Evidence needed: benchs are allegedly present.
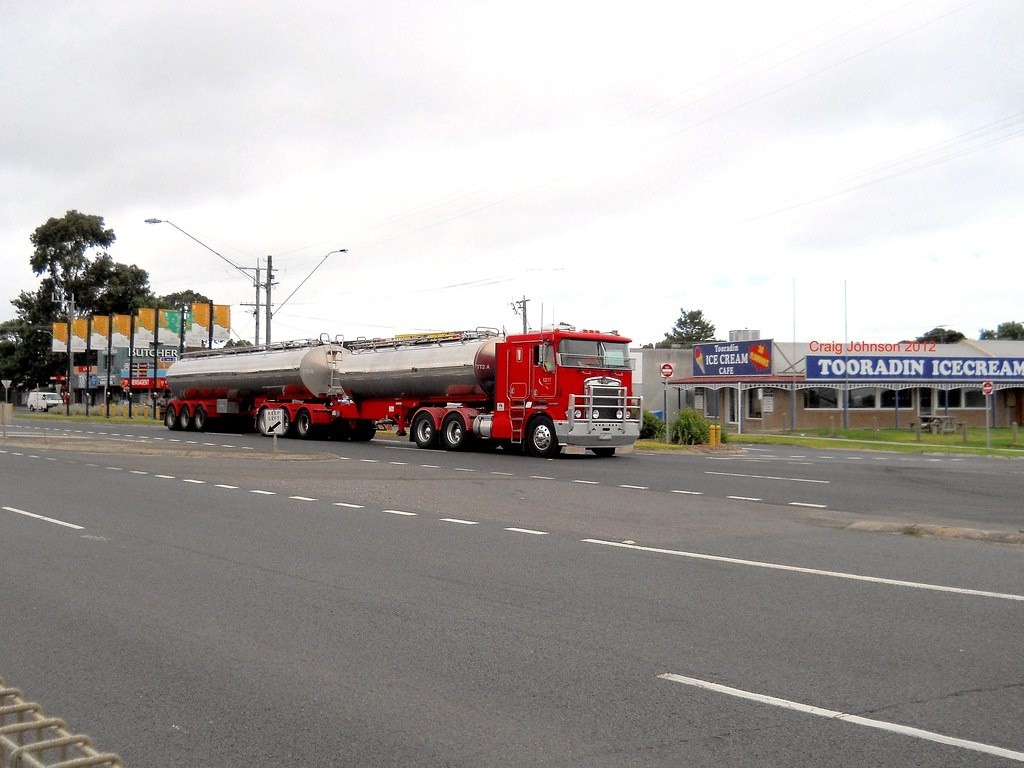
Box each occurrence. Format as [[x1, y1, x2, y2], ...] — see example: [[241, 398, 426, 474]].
[[918, 420, 965, 435]]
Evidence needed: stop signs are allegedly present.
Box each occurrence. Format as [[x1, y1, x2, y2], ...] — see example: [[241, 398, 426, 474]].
[[982, 382, 993, 395], [661, 363, 674, 377]]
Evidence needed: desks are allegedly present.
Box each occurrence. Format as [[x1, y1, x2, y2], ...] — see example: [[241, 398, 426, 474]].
[[919, 415, 956, 435]]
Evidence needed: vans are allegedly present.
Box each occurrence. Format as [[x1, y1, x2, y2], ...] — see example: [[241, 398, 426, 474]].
[[26, 391, 64, 413]]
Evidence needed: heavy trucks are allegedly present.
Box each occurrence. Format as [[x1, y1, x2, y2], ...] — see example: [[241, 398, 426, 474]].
[[160, 323, 644, 459]]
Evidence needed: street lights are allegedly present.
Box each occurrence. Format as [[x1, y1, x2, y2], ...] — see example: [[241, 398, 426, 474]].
[[145, 218, 260, 348], [264, 248, 347, 344]]
